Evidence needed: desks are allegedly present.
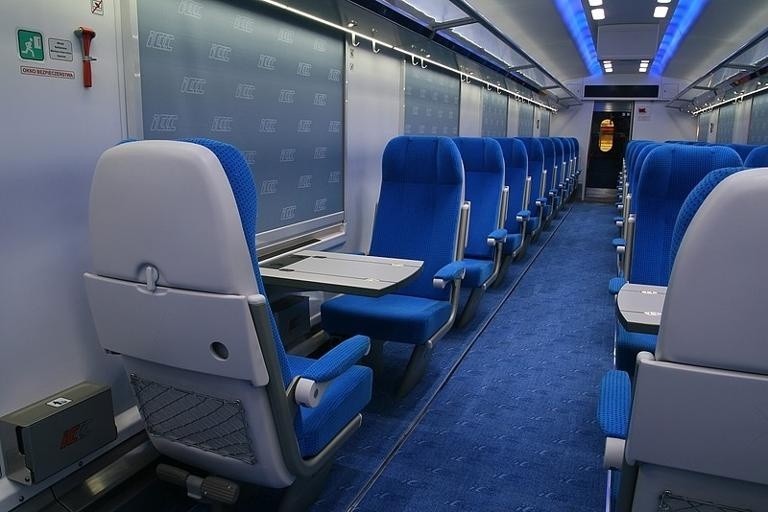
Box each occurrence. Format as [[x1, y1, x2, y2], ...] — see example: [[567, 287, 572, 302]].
[[258, 249, 425, 299]]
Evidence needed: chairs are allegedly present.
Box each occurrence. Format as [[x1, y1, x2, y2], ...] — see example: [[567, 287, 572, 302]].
[[457, 136, 580, 328], [319, 134, 472, 404], [594, 139, 767, 511], [81, 137, 374, 510]]
